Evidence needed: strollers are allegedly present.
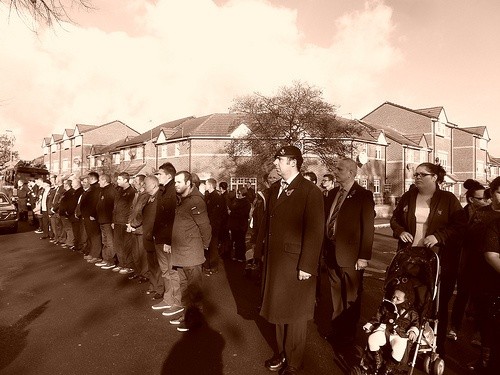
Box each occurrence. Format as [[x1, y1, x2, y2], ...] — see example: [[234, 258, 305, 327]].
[[350, 246, 445, 375]]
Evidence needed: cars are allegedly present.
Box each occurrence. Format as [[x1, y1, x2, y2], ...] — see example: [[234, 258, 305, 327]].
[[0, 192, 18, 233]]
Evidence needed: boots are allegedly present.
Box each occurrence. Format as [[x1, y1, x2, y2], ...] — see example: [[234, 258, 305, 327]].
[[370, 350, 386, 375], [385, 358, 401, 375]]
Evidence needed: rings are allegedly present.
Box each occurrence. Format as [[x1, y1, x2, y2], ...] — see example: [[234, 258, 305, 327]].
[[428, 242, 430, 244]]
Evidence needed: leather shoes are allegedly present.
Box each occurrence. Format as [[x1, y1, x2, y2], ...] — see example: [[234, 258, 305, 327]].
[[264, 352, 287, 372], [282, 366, 298, 375]]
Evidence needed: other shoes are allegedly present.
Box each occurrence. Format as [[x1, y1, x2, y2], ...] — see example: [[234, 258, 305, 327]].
[[144, 289, 156, 294], [176, 322, 189, 331], [138, 278, 149, 283], [204, 268, 219, 277], [34, 230, 134, 274], [169, 315, 185, 324], [152, 301, 171, 310], [161, 307, 184, 316], [153, 294, 163, 301], [127, 276, 140, 279], [246, 263, 259, 270]]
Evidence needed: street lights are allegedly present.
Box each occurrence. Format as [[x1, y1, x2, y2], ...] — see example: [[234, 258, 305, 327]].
[[6, 130, 13, 182]]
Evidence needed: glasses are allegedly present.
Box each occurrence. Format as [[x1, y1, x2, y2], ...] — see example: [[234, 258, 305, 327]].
[[473, 196, 484, 200], [414, 173, 434, 178], [322, 180, 329, 182]]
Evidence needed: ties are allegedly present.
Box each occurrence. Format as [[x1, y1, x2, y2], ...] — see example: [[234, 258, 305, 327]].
[[328, 188, 346, 240], [277, 181, 288, 198]]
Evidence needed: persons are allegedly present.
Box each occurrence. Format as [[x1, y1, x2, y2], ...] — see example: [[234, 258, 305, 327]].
[[363, 287, 419, 375], [303, 172, 317, 185], [126, 174, 149, 284], [259, 146, 325, 375], [321, 173, 334, 195], [191, 173, 269, 269], [390, 164, 467, 358], [30, 172, 102, 263], [94, 174, 117, 269], [112, 172, 134, 273], [153, 163, 185, 316], [320, 158, 376, 345], [451, 175, 500, 370], [16, 179, 31, 222], [170, 171, 211, 332], [141, 176, 165, 300], [268, 168, 281, 186]]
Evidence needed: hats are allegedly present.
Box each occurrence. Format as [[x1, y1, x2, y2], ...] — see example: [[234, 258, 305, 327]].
[[274, 146, 301, 158]]
